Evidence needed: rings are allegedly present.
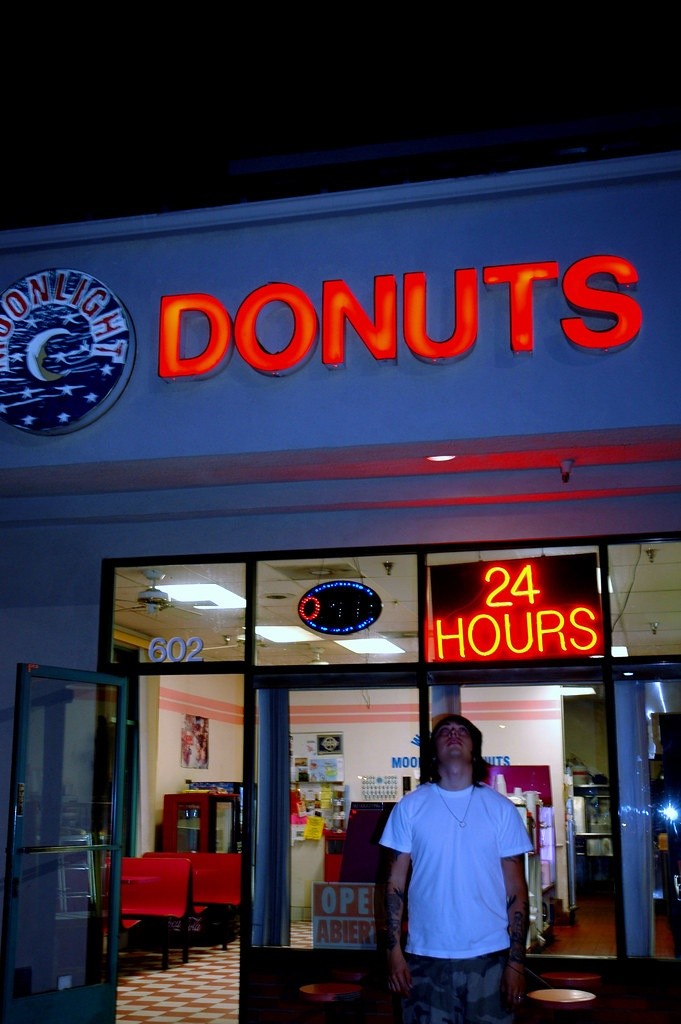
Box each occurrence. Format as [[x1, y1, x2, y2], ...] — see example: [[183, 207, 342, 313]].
[[515, 997, 522, 1000]]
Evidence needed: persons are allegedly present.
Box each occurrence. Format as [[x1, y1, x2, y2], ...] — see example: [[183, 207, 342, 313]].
[[379, 714, 534, 1024]]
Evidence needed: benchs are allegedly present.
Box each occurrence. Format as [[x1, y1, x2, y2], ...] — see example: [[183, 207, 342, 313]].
[[98, 852, 242, 969]]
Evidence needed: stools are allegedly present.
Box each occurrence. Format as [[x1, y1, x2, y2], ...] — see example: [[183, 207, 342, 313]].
[[300, 967, 373, 1024], [525, 971, 602, 1024]]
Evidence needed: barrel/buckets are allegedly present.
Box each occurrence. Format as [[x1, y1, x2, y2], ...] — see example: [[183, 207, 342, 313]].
[[571, 765, 587, 785]]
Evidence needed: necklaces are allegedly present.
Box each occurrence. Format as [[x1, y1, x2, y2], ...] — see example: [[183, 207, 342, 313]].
[[436, 783, 475, 828]]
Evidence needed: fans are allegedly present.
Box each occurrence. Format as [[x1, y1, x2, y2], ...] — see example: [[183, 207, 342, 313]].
[[114, 568, 219, 616]]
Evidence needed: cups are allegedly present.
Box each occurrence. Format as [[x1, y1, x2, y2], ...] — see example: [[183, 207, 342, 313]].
[[494, 774, 508, 797]]
